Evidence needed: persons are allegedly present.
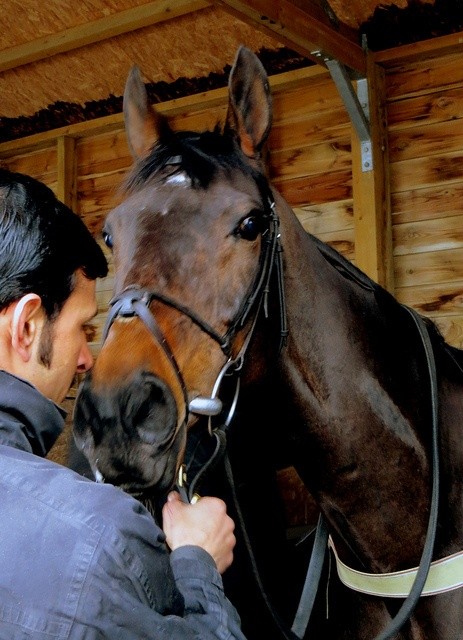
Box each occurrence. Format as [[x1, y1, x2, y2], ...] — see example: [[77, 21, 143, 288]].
[[0, 167, 247, 638]]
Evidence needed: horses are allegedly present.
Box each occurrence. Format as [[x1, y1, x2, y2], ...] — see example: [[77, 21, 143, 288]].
[[68, 41, 463, 640]]
